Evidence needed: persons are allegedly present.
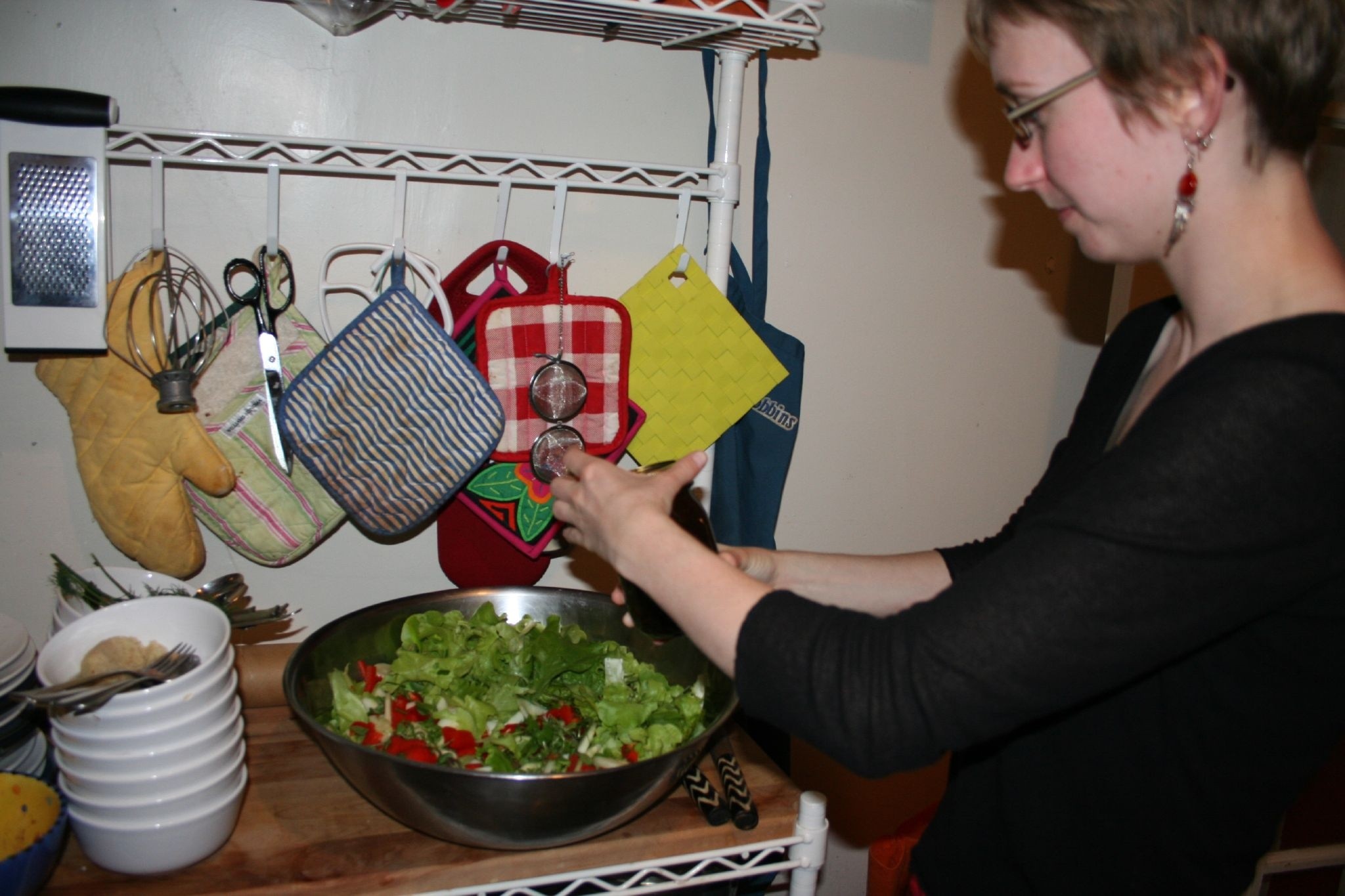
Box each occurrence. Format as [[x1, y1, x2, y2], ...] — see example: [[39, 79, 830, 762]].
[[551, 1, 1345, 896]]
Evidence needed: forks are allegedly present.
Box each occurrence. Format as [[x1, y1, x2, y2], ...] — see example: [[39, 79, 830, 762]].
[[11, 641, 195, 701]]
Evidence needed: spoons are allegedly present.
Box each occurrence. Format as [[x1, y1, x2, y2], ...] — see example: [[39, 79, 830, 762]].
[[216, 584, 249, 605], [192, 573, 244, 600], [72, 653, 201, 715]]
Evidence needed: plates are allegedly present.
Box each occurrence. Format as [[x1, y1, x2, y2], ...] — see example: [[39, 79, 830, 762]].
[[0, 731, 49, 782], [0, 612, 39, 726]]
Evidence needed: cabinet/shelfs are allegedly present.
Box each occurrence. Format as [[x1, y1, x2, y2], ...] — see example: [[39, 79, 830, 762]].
[[41, 0, 833, 896]]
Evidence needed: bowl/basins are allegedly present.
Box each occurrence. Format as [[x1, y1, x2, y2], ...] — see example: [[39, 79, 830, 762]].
[[284, 583, 740, 846], [0, 772, 66, 896], [37, 570, 251, 876]]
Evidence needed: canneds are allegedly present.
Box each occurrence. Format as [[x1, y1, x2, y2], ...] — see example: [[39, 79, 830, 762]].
[[618, 458, 722, 643]]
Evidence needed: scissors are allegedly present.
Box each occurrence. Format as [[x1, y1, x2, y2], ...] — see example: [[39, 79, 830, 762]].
[[226, 245, 294, 476]]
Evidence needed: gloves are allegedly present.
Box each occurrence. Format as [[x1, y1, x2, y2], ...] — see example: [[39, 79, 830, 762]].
[[33, 257, 235, 581]]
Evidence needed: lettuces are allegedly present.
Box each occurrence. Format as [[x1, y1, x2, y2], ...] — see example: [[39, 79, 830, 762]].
[[324, 601, 706, 772]]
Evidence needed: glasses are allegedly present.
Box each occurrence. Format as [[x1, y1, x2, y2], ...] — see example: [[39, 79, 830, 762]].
[[999, 60, 1114, 150]]
